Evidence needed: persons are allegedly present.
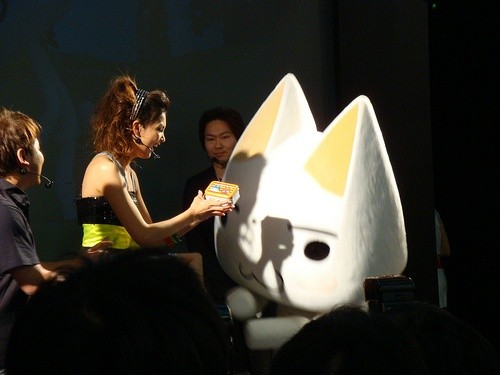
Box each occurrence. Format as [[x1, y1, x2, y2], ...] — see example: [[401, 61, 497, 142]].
[[0, 110, 65, 369], [183, 106, 245, 295], [6, 248, 236, 375], [75, 77, 233, 283], [269, 303, 500, 375]]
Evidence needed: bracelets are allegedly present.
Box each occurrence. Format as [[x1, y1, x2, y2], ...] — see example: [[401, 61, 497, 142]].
[[170, 233, 182, 243], [165, 237, 173, 246]]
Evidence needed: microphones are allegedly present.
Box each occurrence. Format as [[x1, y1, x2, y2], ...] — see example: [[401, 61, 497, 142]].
[[134, 138, 161, 161], [18, 168, 53, 189]]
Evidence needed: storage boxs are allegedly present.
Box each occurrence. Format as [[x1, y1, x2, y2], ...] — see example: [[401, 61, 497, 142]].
[[205, 180, 240, 206]]
